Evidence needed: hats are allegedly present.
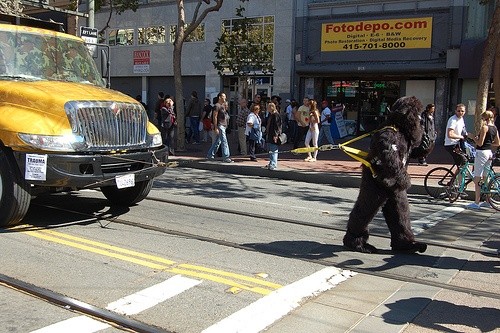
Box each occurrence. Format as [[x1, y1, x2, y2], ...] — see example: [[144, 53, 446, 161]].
[[286, 99, 290, 102]]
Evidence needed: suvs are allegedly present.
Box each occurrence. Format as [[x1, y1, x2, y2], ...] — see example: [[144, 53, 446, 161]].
[[0, 23, 169, 227]]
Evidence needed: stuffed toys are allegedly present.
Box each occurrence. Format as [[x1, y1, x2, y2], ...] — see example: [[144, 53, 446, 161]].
[[343, 95, 430, 253]]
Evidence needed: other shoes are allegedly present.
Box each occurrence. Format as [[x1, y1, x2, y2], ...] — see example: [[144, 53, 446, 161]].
[[447, 182, 458, 188], [185, 139, 189, 143], [454, 191, 468, 197], [465, 203, 480, 209], [264, 165, 277, 170], [418, 162, 428, 166], [480, 202, 490, 206], [192, 142, 200, 145], [304, 156, 316, 162], [223, 157, 234, 163], [207, 154, 215, 160], [251, 158, 258, 162]]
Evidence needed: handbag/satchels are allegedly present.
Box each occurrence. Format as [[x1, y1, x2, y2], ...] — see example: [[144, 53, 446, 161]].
[[328, 118, 332, 123], [278, 133, 287, 145], [199, 120, 203, 131], [185, 118, 190, 127], [203, 118, 213, 131], [250, 127, 260, 140]]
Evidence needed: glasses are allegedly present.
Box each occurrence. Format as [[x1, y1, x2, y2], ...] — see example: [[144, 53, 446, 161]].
[[258, 97, 261, 98], [457, 110, 465, 113], [481, 117, 484, 119]]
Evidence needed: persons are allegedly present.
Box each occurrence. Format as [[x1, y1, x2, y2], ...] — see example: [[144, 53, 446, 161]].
[[153, 92, 310, 149], [160, 98, 177, 156], [318, 100, 334, 147], [211, 96, 231, 157], [244, 104, 262, 162], [444, 104, 468, 199], [206, 91, 235, 163], [135, 95, 148, 115], [184, 90, 201, 145], [264, 102, 282, 170], [465, 110, 500, 208], [304, 100, 321, 162], [409, 104, 438, 165], [235, 99, 251, 157]]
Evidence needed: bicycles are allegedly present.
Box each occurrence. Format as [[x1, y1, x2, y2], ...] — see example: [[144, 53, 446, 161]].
[[424, 136, 500, 212]]
